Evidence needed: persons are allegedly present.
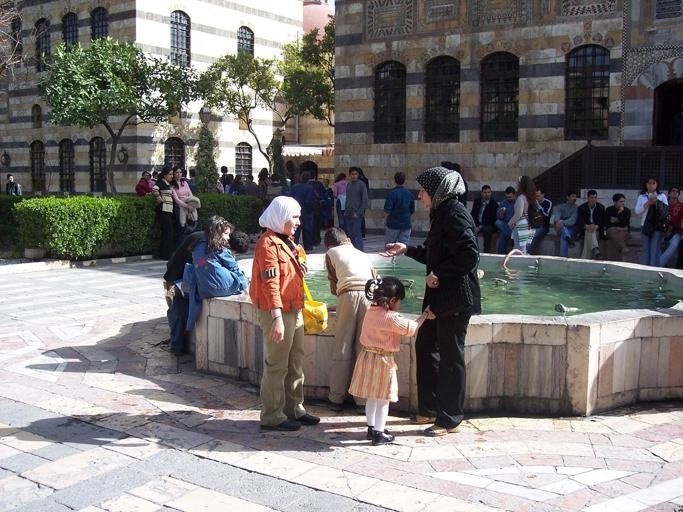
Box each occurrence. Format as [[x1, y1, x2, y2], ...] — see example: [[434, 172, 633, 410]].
[[163, 215, 250, 356], [348, 277, 435, 446], [186, 214, 249, 331], [377, 166, 482, 437], [6, 174, 22, 196], [249, 196, 321, 431], [323, 226, 376, 414]]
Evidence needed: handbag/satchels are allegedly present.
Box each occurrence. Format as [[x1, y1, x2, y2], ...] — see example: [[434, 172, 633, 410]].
[[302, 299, 328, 335]]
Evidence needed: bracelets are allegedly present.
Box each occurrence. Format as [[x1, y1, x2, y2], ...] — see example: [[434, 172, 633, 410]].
[[272, 316, 280, 323], [424, 311, 429, 319]]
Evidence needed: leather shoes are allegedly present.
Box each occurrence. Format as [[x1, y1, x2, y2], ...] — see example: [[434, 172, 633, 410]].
[[372, 429, 395, 445], [260, 418, 301, 431], [365, 424, 389, 440], [296, 413, 320, 425]]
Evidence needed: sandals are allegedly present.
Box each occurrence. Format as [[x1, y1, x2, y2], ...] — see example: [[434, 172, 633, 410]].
[[409, 413, 436, 425], [423, 422, 462, 437]]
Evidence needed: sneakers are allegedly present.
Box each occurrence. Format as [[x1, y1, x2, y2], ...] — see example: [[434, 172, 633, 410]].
[[593, 248, 602, 258]]
